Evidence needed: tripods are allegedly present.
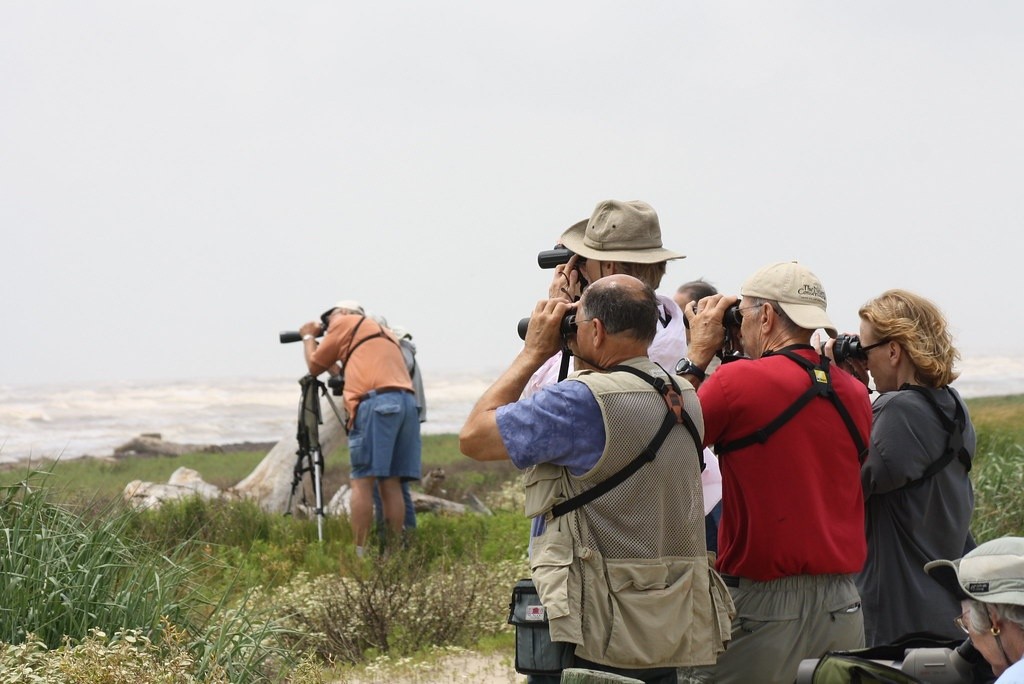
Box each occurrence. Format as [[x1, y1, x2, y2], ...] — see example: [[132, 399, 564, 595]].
[[286, 376, 350, 542]]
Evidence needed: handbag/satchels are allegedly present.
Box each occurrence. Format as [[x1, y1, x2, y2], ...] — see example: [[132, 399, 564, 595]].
[[507, 579, 577, 677]]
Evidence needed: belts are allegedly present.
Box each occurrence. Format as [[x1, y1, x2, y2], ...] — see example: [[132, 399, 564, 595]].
[[358, 386, 416, 403]]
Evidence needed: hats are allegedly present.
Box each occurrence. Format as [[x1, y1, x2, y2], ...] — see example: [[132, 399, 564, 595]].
[[560, 199, 687, 264], [320, 303, 365, 327], [923, 536, 1024, 605], [741, 261, 838, 339]]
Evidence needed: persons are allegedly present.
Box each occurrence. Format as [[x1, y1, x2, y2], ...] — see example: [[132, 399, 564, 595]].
[[672, 259, 874, 684], [459, 273, 737, 684], [814, 287, 977, 662], [362, 313, 427, 559], [298, 306, 422, 565], [673, 277, 717, 351], [535, 199, 718, 541], [922, 535, 1024, 684]]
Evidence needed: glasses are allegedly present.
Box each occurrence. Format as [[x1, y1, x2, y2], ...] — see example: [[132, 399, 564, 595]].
[[952, 610, 972, 637], [732, 303, 783, 325]]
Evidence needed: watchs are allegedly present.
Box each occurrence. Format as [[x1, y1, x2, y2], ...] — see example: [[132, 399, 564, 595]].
[[302, 334, 315, 340], [674, 355, 707, 382]]
